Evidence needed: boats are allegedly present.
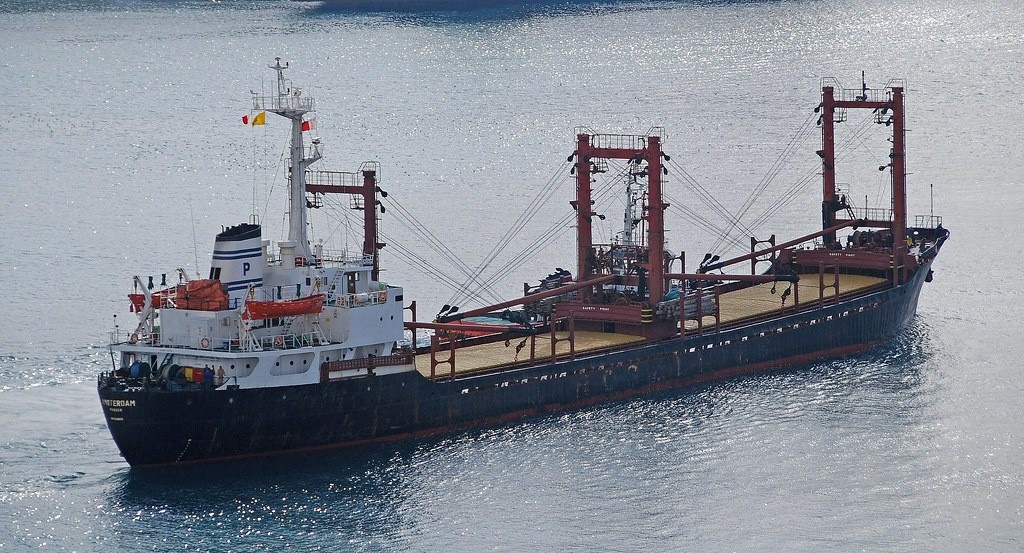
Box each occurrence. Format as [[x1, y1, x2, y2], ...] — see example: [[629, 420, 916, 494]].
[[97, 55, 951, 470]]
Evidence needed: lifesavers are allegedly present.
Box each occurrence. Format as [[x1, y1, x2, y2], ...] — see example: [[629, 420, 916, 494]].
[[201, 337, 210, 348], [379, 292, 386, 301], [131, 334, 138, 343]]
